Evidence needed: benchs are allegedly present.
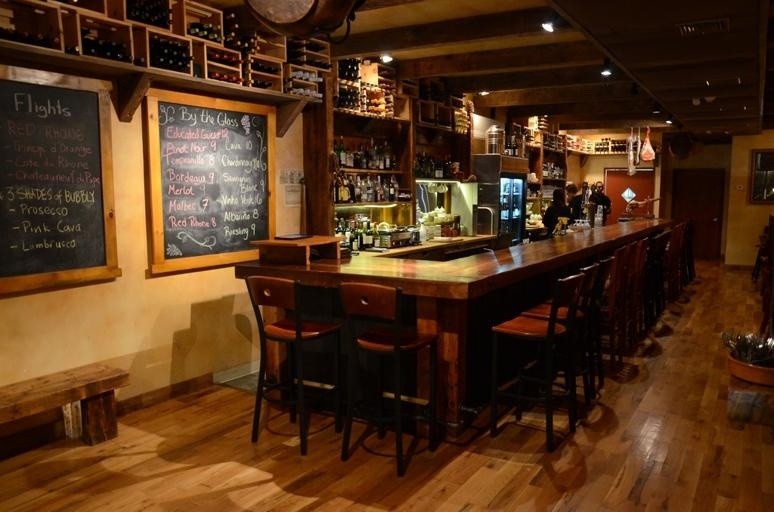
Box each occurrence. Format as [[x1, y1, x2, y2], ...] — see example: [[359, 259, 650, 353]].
[[0, 361, 134, 450]]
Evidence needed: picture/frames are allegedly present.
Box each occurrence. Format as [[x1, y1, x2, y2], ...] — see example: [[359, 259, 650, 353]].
[[746, 147, 774, 206]]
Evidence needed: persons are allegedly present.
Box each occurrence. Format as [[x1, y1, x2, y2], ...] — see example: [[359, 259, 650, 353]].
[[564, 183, 583, 220], [542, 189, 574, 239], [578, 181, 612, 228]]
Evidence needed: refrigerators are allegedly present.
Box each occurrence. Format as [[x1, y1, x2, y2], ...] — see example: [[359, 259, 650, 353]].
[[472, 156, 530, 250]]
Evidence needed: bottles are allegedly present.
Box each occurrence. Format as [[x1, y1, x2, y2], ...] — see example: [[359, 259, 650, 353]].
[[333, 132, 401, 202], [419, 215, 465, 241], [414, 149, 461, 179], [505, 133, 518, 157], [333, 216, 384, 256], [0, 0, 400, 119]]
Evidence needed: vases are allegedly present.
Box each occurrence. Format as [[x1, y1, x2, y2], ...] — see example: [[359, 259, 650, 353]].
[[728, 356, 774, 402]]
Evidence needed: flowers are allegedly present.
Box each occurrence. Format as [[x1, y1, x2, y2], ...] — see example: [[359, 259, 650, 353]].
[[723, 329, 774, 367]]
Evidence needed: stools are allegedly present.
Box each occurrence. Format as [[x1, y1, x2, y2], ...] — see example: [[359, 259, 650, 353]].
[[335, 277, 441, 482], [550, 220, 694, 391], [485, 272, 585, 454], [241, 273, 344, 457], [522, 261, 598, 413]]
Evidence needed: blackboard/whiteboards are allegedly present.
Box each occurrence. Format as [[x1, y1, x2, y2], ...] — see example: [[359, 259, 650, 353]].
[[0, 64, 123, 294], [142, 88, 276, 274]]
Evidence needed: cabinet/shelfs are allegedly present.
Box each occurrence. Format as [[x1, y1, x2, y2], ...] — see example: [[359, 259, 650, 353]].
[[2, 2, 332, 111], [334, 60, 656, 244]]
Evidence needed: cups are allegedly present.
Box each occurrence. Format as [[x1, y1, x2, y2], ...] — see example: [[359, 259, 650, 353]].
[[572, 219, 594, 235]]
